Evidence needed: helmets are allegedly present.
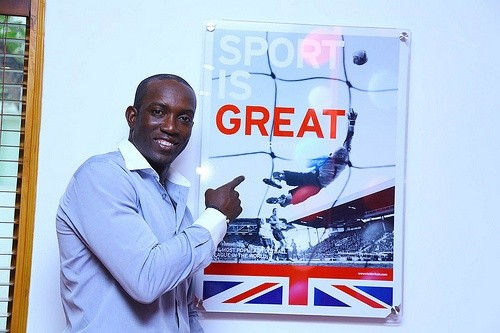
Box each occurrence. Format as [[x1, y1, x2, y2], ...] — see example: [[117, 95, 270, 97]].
[[331, 146, 349, 164]]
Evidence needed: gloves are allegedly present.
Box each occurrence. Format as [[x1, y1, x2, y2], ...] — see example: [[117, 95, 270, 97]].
[[347, 108, 358, 127]]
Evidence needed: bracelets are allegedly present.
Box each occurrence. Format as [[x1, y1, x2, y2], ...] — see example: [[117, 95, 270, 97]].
[[204, 204, 232, 228]]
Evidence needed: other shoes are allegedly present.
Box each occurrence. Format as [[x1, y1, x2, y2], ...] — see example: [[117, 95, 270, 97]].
[[263, 178, 283, 189], [266, 194, 287, 205]]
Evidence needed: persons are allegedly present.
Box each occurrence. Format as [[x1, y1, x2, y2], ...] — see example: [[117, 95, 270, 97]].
[[263, 108, 357, 264], [56, 74, 245, 333]]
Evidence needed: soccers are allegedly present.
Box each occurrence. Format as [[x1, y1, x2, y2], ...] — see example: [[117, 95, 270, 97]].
[[352, 49, 368, 65]]
